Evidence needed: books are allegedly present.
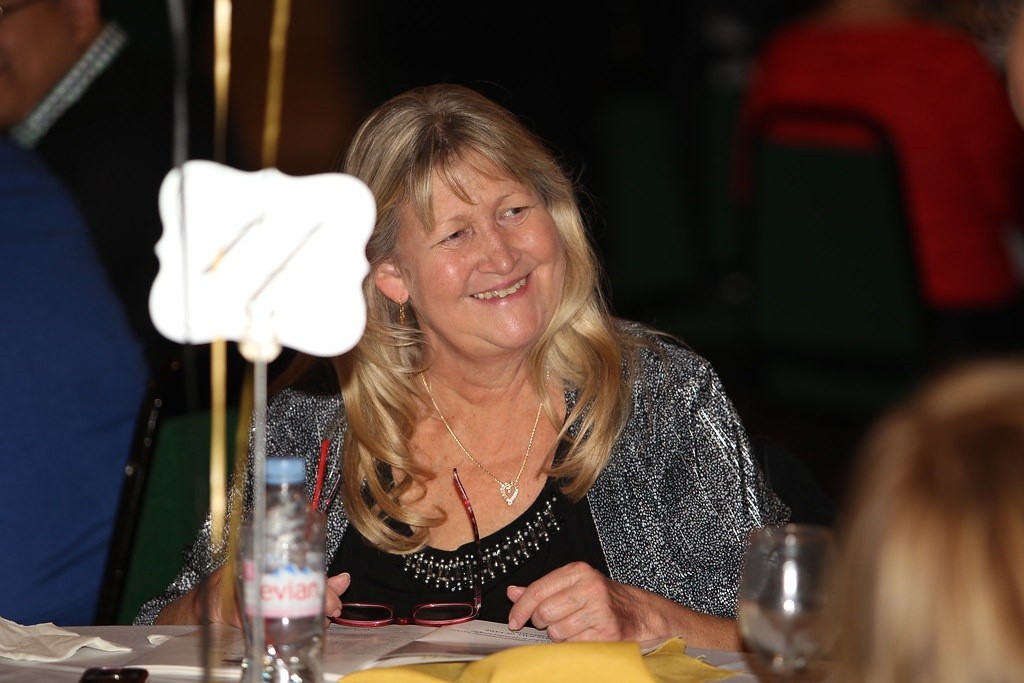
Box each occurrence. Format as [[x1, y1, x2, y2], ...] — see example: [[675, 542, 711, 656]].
[[123, 622, 560, 683]]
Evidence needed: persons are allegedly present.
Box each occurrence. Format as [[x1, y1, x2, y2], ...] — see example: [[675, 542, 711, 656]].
[[739, 0, 1024, 348], [829, 359, 1024, 682], [1, 0, 358, 621], [133, 86, 790, 652]]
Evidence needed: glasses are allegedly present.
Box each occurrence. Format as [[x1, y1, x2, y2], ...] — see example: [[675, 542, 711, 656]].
[[322, 466, 483, 626]]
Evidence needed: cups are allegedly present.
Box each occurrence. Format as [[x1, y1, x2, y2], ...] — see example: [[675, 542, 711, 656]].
[[736, 527, 844, 683]]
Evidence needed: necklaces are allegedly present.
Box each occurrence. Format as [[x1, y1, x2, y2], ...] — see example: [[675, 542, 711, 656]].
[[418, 367, 551, 504]]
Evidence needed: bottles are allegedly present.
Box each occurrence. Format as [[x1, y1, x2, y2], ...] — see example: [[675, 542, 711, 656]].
[[240, 457, 327, 683]]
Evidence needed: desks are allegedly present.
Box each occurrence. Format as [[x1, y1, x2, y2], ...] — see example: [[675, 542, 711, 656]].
[[0, 615, 759, 683]]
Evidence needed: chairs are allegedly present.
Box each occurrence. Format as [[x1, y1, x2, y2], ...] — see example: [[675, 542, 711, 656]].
[[594, 85, 928, 413], [92, 370, 332, 623]]
